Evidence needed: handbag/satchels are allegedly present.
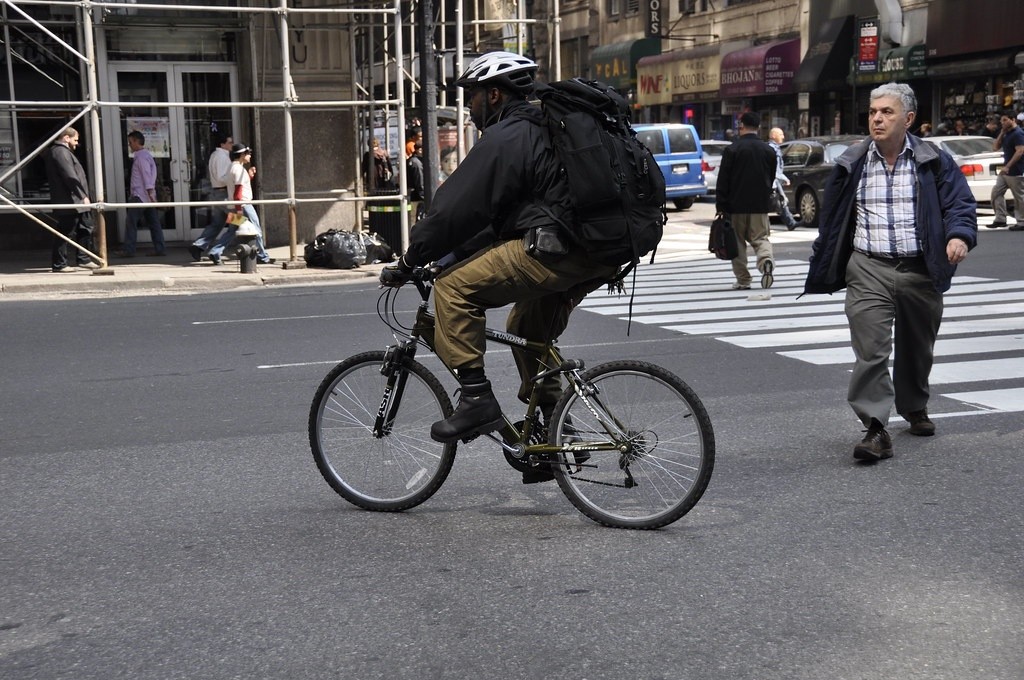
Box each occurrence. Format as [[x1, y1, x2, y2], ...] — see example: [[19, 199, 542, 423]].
[[523, 224, 571, 261], [768, 186, 786, 214], [708, 213, 739, 260]]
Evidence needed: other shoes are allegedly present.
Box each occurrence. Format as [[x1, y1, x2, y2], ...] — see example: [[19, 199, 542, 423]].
[[77, 260, 99, 269], [731, 281, 751, 290], [985, 221, 1008, 228], [52, 265, 78, 274], [1009, 224, 1024, 232], [113, 247, 136, 256], [208, 252, 224, 265], [759, 259, 773, 287], [146, 248, 167, 256], [187, 244, 203, 261], [788, 219, 808, 230], [258, 258, 276, 263]]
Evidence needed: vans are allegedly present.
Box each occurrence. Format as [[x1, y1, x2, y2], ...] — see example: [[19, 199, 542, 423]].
[[359, 110, 399, 160], [629, 122, 709, 211]]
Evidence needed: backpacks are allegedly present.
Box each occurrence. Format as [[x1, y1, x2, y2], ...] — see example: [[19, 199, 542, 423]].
[[491, 76, 669, 271]]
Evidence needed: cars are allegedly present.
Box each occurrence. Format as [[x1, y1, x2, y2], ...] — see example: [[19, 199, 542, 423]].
[[699, 140, 733, 194], [919, 135, 1016, 217], [778, 134, 868, 228]]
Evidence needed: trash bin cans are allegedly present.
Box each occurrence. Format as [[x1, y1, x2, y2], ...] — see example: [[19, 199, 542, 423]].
[[367, 189, 411, 256]]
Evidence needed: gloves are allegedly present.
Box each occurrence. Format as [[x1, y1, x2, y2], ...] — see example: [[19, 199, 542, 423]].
[[379, 262, 411, 289]]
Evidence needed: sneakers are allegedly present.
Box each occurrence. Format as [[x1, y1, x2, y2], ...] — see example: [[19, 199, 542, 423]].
[[853, 426, 896, 460], [906, 410, 935, 435]]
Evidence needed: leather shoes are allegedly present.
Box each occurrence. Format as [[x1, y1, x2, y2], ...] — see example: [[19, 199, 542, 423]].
[[429, 380, 507, 441], [522, 413, 594, 484]]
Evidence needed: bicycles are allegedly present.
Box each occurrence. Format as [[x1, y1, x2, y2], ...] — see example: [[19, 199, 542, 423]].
[[309, 266, 716, 530]]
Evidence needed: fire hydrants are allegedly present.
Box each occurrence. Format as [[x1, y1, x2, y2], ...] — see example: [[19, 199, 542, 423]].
[[234, 220, 261, 275]]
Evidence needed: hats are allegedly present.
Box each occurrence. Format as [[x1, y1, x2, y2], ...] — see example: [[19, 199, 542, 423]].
[[233, 142, 255, 155]]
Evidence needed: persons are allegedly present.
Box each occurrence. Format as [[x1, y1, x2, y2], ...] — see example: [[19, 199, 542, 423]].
[[794, 82, 978, 456], [112, 131, 168, 257], [920, 102, 1024, 232], [715, 111, 778, 289], [206, 144, 276, 264], [767, 128, 803, 231], [360, 126, 425, 229], [438, 146, 458, 183], [379, 51, 615, 462], [52, 127, 97, 273], [187, 134, 234, 260]]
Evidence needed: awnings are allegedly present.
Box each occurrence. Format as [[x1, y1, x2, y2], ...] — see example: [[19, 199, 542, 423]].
[[718, 36, 800, 100]]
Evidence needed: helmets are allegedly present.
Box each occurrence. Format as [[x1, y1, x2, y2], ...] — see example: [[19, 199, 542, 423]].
[[454, 53, 540, 92]]
